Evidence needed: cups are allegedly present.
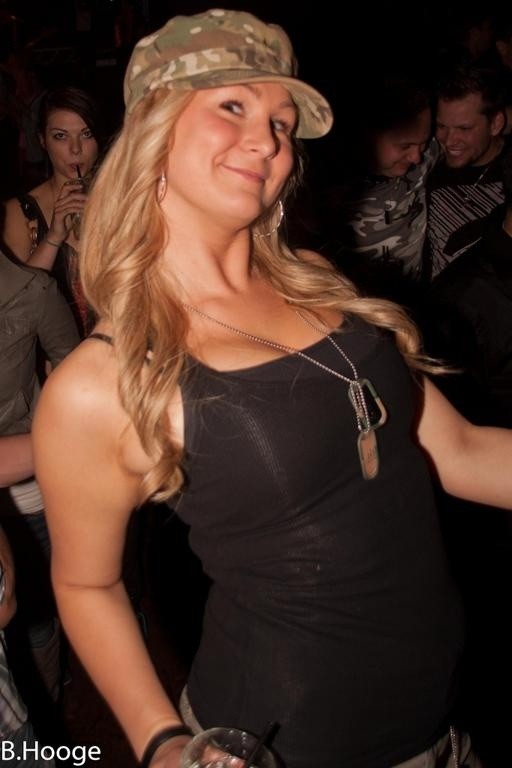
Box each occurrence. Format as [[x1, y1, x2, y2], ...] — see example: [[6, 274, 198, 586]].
[[178, 725, 278, 768], [62, 176, 94, 243]]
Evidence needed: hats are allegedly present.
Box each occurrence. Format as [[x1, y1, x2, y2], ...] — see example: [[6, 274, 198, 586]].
[[121, 8, 335, 142]]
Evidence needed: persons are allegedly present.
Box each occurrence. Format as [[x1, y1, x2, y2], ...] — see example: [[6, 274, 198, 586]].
[[1, 0, 512, 768]]
